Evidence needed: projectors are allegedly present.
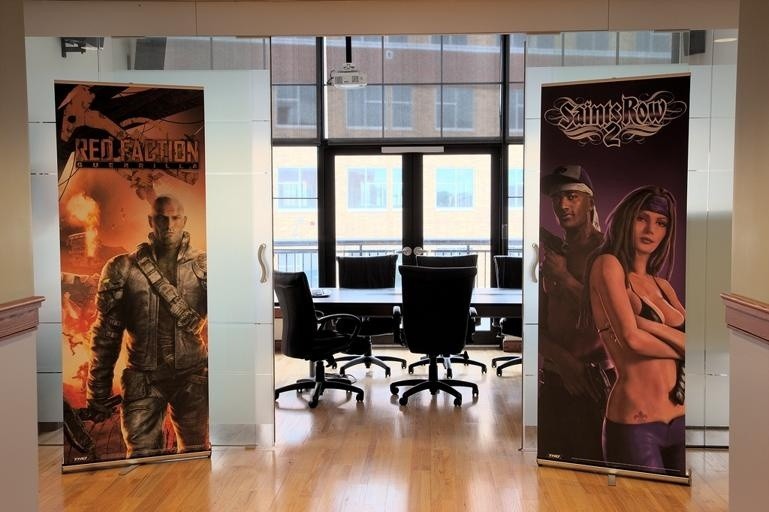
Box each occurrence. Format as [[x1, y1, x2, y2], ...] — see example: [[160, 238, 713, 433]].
[[330, 72, 368, 88]]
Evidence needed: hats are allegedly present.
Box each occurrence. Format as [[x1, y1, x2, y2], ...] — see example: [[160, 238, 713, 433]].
[[543, 164, 594, 198]]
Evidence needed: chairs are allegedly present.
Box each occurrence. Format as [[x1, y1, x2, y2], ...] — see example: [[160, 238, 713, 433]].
[[274, 254, 523, 409]]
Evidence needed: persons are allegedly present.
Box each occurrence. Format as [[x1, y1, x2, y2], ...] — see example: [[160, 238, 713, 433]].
[[61, 275, 92, 307], [85, 195, 207, 458], [580, 185, 685, 480], [539, 161, 619, 465]]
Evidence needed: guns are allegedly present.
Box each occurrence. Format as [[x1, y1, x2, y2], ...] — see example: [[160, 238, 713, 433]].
[[581, 358, 613, 420], [540, 226, 570, 257]]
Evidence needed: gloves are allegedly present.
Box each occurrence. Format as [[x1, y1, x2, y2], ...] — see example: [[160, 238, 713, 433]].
[[86, 398, 115, 424]]
